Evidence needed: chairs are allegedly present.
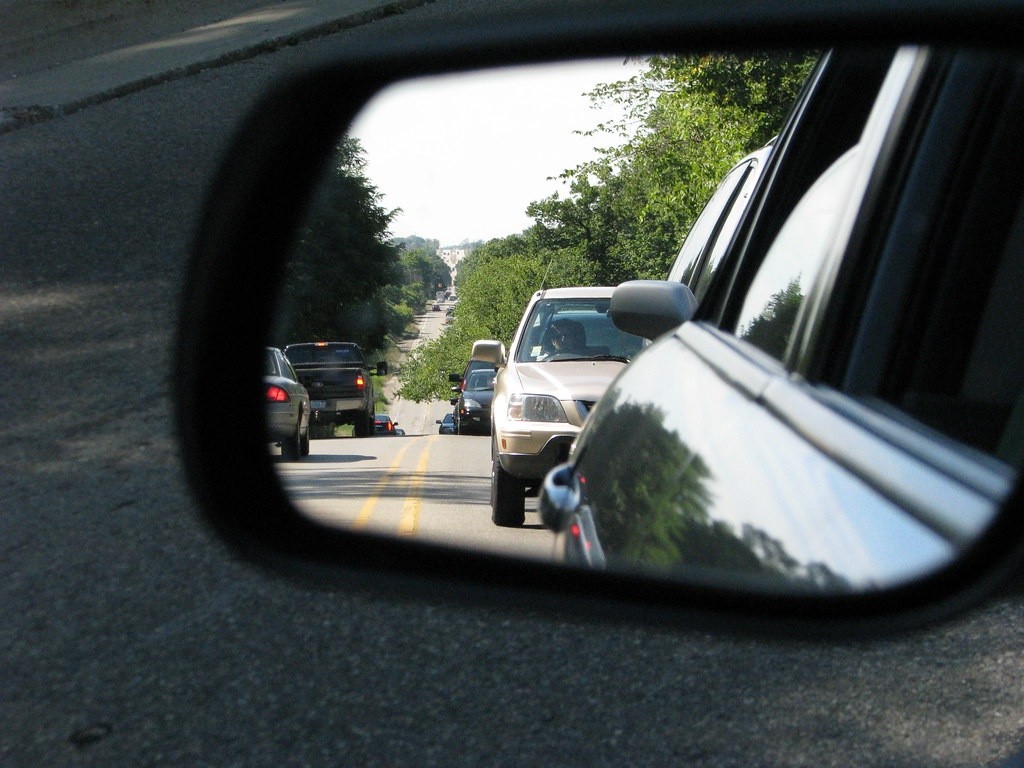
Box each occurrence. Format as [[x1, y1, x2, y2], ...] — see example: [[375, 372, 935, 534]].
[[475, 378, 486, 387], [541, 318, 586, 356]]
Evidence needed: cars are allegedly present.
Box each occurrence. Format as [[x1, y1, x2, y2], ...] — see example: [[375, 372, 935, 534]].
[[448, 360, 498, 436], [436, 413, 455, 434], [432, 289, 458, 326], [395, 429, 405, 436], [375, 414, 398, 435], [261, 347, 310, 461]]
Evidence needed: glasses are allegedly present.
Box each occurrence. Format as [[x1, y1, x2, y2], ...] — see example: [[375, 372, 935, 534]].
[[550, 334, 571, 340]]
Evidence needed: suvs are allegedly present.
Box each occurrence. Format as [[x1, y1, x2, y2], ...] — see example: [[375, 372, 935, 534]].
[[638, 137, 784, 355], [471, 287, 655, 527]]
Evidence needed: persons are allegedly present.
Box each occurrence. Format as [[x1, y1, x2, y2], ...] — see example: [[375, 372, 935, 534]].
[[534, 319, 603, 361]]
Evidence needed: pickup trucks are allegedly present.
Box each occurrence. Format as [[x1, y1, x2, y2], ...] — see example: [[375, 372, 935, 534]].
[[283, 343, 387, 437]]
